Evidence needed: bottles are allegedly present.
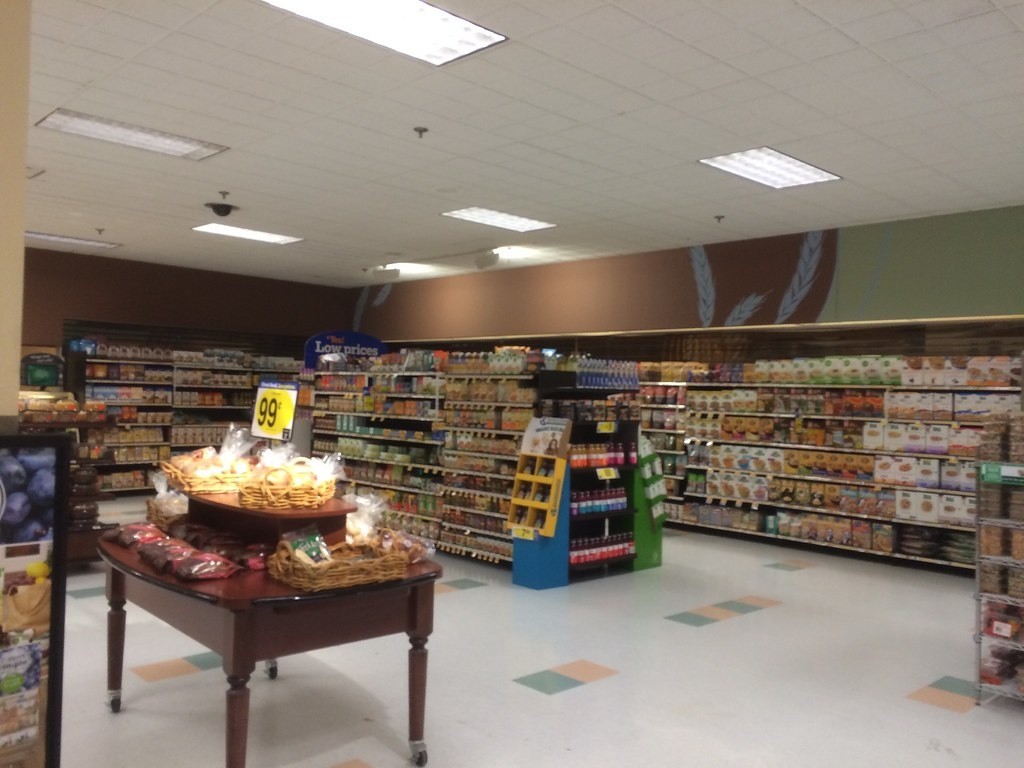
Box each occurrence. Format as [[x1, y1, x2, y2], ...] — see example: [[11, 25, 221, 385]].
[[445, 492, 511, 515], [568, 351, 639, 391], [534, 485, 550, 502], [443, 509, 511, 536], [521, 459, 533, 474], [568, 487, 627, 515], [531, 511, 545, 528], [514, 506, 529, 525], [515, 481, 531, 501], [537, 459, 553, 478], [440, 525, 513, 559], [568, 442, 638, 468], [446, 474, 511, 494], [569, 532, 636, 565]]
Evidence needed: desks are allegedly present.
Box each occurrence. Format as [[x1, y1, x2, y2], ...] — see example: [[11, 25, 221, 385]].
[[94, 533, 442, 768]]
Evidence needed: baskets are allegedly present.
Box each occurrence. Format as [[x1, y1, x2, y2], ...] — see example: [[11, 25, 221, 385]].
[[239, 458, 336, 509], [267, 528, 408, 592], [160, 460, 254, 495]]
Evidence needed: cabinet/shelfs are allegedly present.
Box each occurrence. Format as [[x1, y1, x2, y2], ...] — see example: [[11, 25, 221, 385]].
[[970, 456, 1024, 705], [310, 373, 640, 583], [67, 356, 303, 494], [506, 451, 568, 537], [641, 383, 1024, 572]]
